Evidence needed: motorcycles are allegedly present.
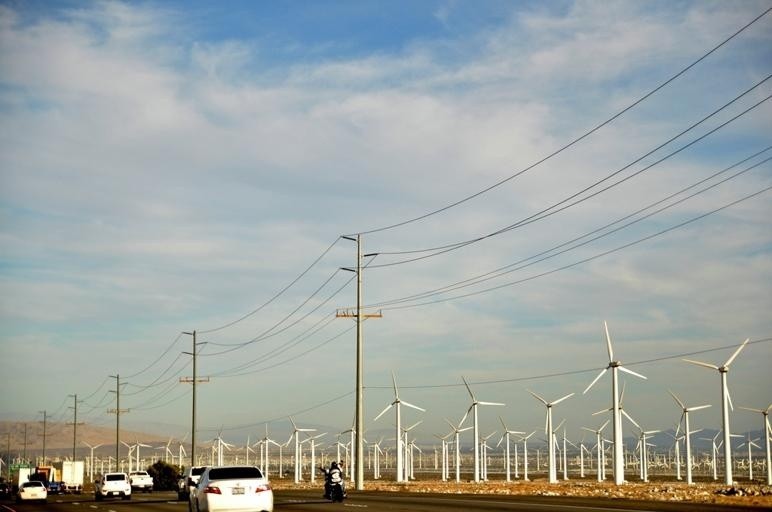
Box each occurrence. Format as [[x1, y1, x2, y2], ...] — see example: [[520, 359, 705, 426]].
[[319, 466, 347, 503]]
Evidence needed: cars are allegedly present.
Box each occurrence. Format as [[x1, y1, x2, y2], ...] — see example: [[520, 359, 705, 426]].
[[94, 471, 154, 501], [177, 466, 274, 512], [0, 481, 49, 504]]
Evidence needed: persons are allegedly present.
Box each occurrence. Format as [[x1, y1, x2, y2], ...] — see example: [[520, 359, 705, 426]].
[[325, 460, 347, 501]]
